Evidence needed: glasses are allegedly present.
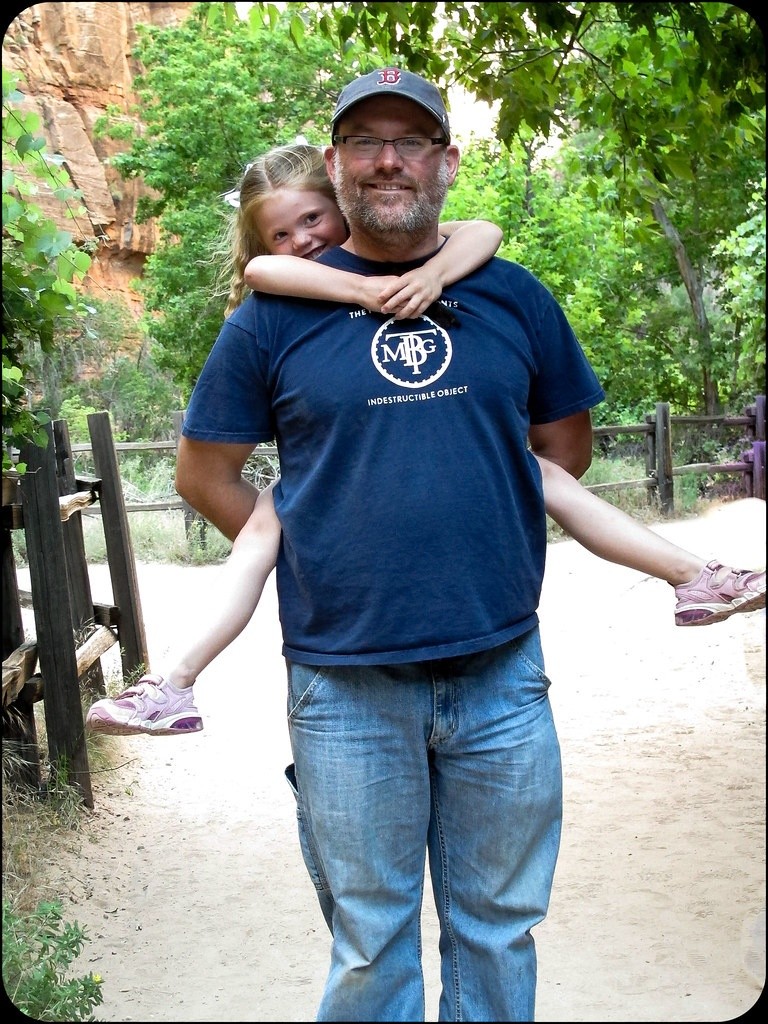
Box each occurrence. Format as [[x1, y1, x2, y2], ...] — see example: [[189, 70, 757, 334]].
[[334, 134, 449, 162]]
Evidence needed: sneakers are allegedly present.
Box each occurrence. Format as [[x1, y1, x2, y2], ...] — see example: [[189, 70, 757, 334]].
[[667, 560, 766, 626], [86, 675, 204, 736]]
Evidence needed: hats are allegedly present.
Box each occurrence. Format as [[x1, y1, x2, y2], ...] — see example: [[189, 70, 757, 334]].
[[330, 67, 451, 144]]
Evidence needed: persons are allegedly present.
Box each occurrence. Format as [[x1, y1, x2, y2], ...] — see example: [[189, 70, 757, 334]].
[[174, 67, 606, 1023], [85, 142, 767, 737]]
